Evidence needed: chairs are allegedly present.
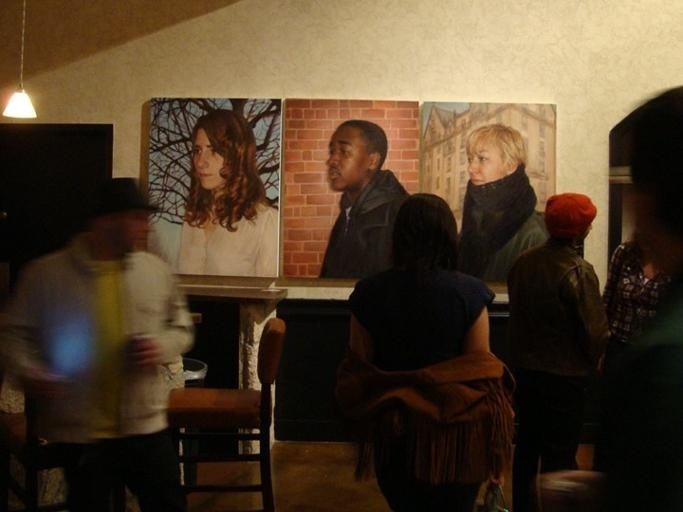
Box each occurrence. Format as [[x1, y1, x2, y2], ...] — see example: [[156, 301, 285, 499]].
[[0, 375, 71, 511], [165, 314, 289, 509]]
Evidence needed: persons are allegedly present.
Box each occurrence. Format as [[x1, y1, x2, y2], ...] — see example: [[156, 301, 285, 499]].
[[0, 177, 196, 512], [318, 120, 412, 277], [176, 110, 280, 276], [507, 192, 614, 512], [347, 194, 517, 512], [458, 125, 551, 281], [605, 84, 683, 512]]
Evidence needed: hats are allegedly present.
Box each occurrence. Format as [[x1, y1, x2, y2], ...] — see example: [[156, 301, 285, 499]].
[[544, 192, 598, 237], [87, 177, 159, 215]]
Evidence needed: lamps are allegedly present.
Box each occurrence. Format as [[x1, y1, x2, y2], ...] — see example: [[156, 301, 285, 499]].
[[0, 0, 37, 121]]
